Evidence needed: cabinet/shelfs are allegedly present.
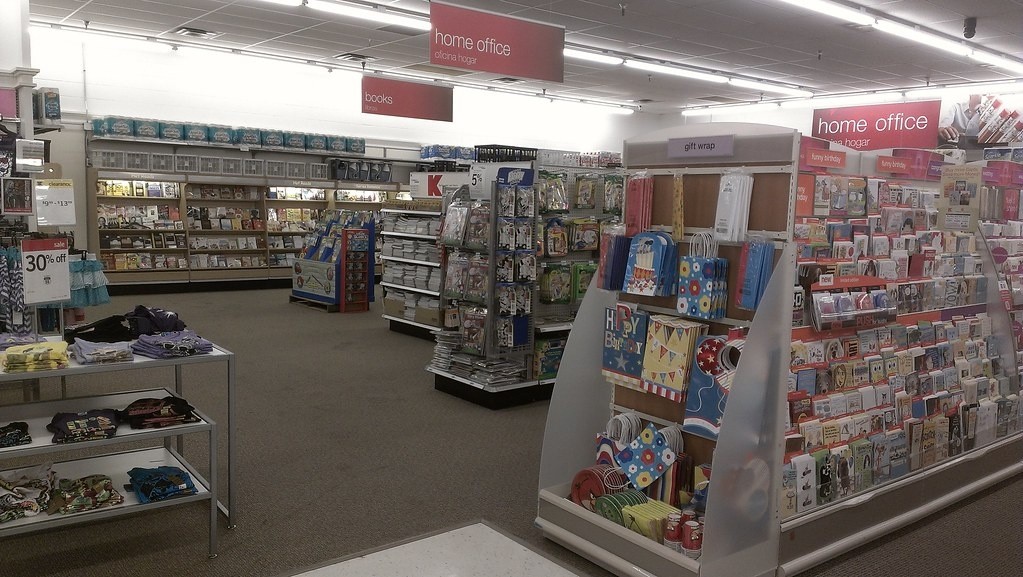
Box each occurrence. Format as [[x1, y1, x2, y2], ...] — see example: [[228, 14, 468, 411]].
[[0, 86, 1023, 577]]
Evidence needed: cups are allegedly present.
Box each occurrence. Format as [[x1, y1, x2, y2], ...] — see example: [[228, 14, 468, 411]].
[[664, 509, 705, 560]]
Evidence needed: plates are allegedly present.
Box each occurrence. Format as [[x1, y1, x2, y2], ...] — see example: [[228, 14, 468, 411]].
[[571, 462, 648, 529]]
